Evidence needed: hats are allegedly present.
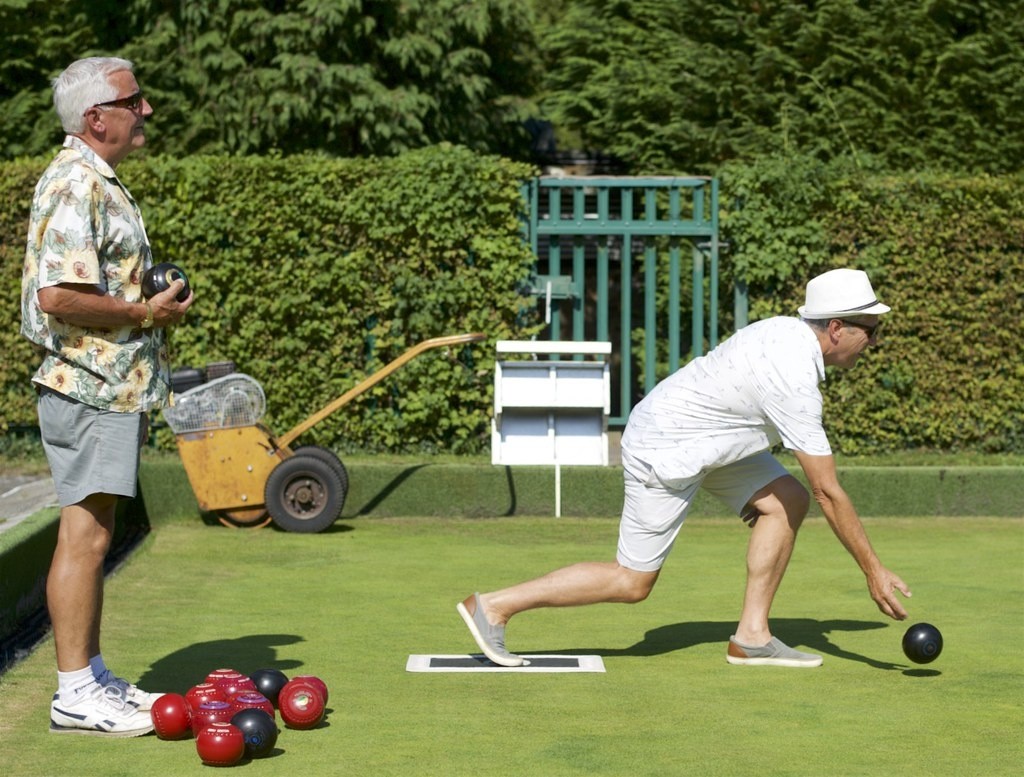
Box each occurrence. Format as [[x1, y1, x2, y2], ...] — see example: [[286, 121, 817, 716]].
[[799, 268, 891, 318]]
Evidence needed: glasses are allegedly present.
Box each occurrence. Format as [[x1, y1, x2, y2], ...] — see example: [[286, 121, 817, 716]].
[[83, 91, 142, 116]]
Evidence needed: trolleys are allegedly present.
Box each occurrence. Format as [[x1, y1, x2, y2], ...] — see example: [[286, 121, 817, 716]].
[[164, 332, 489, 534]]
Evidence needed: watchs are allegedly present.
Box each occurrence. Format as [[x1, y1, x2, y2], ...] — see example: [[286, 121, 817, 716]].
[[139, 302, 155, 329]]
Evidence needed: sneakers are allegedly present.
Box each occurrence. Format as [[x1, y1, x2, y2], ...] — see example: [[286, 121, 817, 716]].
[[456, 590, 522, 666], [49, 669, 167, 739], [726, 634, 823, 667]]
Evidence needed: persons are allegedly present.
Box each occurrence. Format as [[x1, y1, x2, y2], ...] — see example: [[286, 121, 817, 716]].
[[457, 267, 914, 668], [20, 55, 194, 736]]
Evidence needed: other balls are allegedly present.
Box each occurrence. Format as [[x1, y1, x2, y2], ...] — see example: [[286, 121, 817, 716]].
[[902, 621, 943, 665], [150, 667, 329, 766], [142, 262, 190, 304]]
